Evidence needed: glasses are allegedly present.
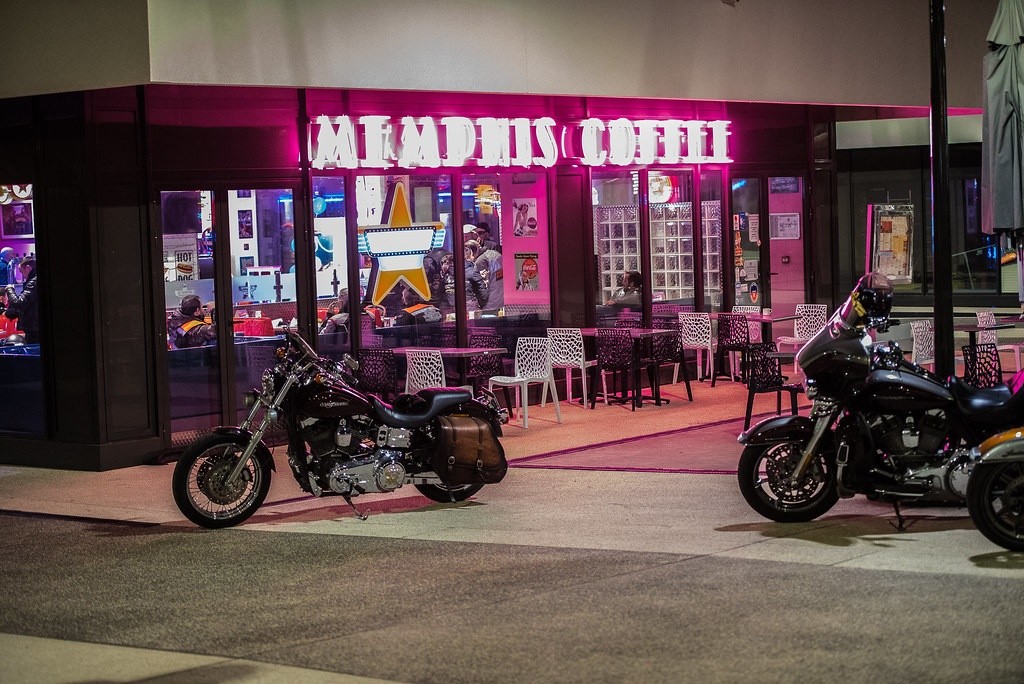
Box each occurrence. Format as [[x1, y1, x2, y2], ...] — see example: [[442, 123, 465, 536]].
[[477, 231, 488, 235]]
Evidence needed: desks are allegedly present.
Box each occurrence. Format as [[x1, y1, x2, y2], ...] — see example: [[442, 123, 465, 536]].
[[361, 346, 514, 418], [579, 328, 693, 408], [929, 324, 1016, 386], [698, 312, 802, 383]]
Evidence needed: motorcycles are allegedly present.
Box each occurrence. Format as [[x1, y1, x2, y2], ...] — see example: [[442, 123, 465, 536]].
[[737, 272, 1024, 549], [172, 328, 509, 528]]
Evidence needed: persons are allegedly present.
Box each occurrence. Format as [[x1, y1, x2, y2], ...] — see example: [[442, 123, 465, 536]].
[[168, 295, 216, 350], [604, 271, 642, 307], [0, 247, 37, 344], [318, 288, 349, 334], [392, 222, 503, 327]]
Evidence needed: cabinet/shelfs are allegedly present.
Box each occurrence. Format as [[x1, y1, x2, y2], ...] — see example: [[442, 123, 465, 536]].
[[596, 201, 721, 310]]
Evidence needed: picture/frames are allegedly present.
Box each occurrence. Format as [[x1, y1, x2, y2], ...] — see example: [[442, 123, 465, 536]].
[[0, 200, 35, 240]]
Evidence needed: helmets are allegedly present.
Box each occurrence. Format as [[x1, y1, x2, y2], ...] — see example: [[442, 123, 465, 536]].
[[857, 272, 894, 319]]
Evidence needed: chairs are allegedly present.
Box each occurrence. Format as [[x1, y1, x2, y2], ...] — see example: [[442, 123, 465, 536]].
[[910, 311, 1024, 390], [244, 346, 278, 437], [359, 304, 827, 428]]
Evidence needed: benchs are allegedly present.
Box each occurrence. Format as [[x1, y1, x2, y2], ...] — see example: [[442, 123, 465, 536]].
[[596, 296, 711, 315], [318, 307, 382, 327], [233, 307, 275, 338]]
